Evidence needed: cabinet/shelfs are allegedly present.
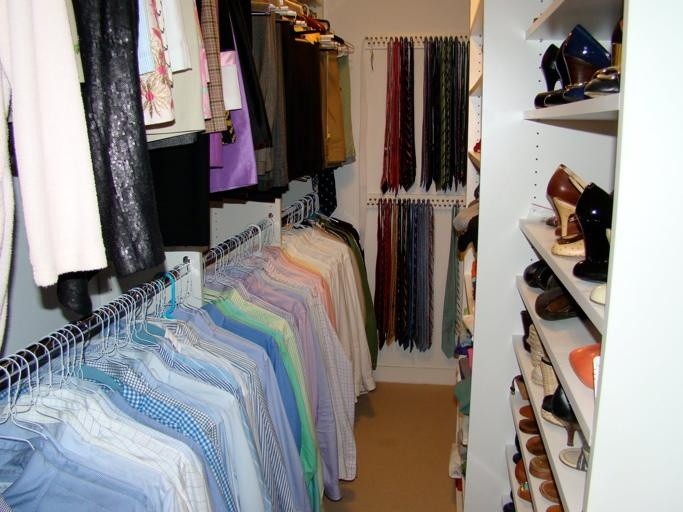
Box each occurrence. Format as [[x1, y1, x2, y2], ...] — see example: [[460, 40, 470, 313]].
[[452, 2, 681, 510]]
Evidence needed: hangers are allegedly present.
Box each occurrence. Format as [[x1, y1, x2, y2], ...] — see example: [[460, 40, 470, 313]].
[[252, 0, 356, 57], [0, 191, 341, 457]]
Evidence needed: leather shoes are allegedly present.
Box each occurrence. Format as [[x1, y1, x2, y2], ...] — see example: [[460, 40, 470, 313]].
[[523, 258, 554, 291], [521, 310, 561, 397], [546, 215, 607, 306], [534, 286, 583, 321], [534, 66, 623, 109], [541, 395, 580, 432]]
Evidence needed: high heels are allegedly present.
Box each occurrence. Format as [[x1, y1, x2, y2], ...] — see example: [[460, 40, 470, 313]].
[[542, 24, 611, 92], [550, 384, 578, 447], [546, 164, 584, 237], [575, 182, 612, 259]]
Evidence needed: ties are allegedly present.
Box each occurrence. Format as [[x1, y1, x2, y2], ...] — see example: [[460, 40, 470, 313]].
[[419, 35, 469, 193], [440, 204, 458, 358], [373, 198, 434, 352], [380, 37, 416, 197]]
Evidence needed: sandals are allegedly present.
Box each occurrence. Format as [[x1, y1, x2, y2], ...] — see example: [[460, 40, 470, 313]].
[[558, 445, 589, 472]]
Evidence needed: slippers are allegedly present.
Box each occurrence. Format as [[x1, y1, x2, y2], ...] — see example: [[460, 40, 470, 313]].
[[525, 436, 545, 456], [518, 419, 539, 434], [519, 405, 534, 419], [545, 504, 563, 512], [538, 480, 560, 505], [528, 455, 553, 481]]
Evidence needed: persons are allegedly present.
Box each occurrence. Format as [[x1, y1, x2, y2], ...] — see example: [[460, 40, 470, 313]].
[[455, 186, 478, 262]]
